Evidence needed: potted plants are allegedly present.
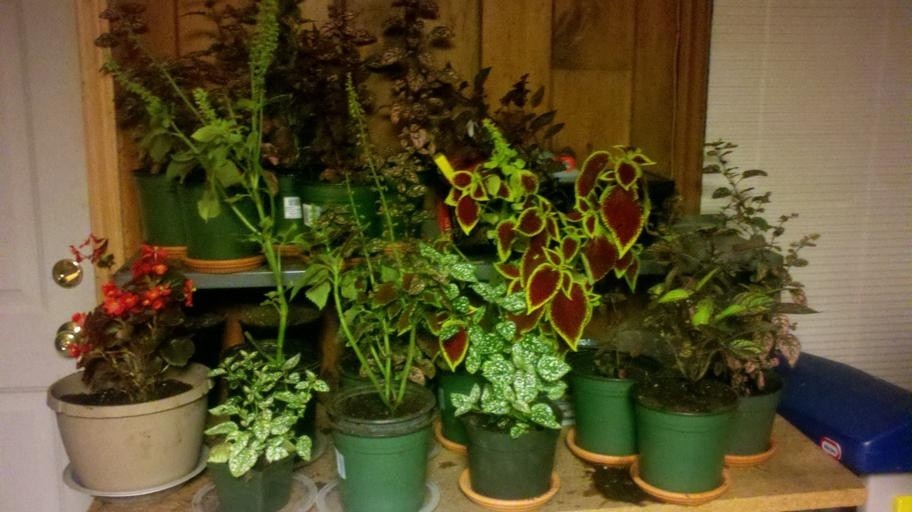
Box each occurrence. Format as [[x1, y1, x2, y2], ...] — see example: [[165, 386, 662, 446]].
[[94, 2, 473, 510], [429, 67, 823, 500]]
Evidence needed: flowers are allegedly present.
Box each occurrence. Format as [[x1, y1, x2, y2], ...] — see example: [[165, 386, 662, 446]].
[[71, 234, 194, 398]]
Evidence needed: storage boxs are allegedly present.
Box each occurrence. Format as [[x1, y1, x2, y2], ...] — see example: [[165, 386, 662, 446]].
[[779, 348, 912, 474], [852, 471, 912, 512]]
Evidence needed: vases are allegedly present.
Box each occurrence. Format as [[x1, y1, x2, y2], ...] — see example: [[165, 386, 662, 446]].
[[48, 361, 212, 492]]
